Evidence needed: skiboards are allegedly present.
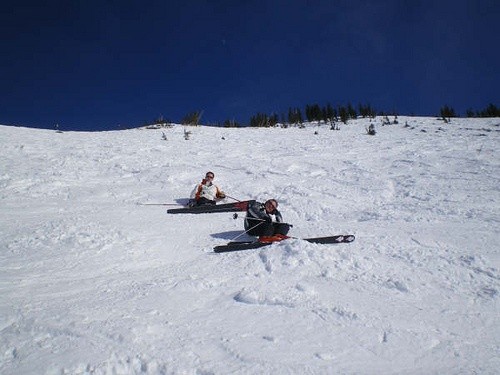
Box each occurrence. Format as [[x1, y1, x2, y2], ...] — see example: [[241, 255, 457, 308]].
[[214, 235, 355, 253], [167, 200, 256, 214]]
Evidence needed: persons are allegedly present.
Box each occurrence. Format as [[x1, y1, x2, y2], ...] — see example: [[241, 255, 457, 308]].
[[188, 172, 226, 208], [244, 199, 290, 244]]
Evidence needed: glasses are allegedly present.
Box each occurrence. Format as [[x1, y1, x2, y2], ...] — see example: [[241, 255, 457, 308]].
[[206, 176, 213, 179], [268, 200, 275, 209]]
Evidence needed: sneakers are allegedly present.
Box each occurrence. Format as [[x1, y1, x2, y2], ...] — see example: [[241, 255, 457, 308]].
[[259, 236, 282, 244], [275, 233, 289, 239]]
